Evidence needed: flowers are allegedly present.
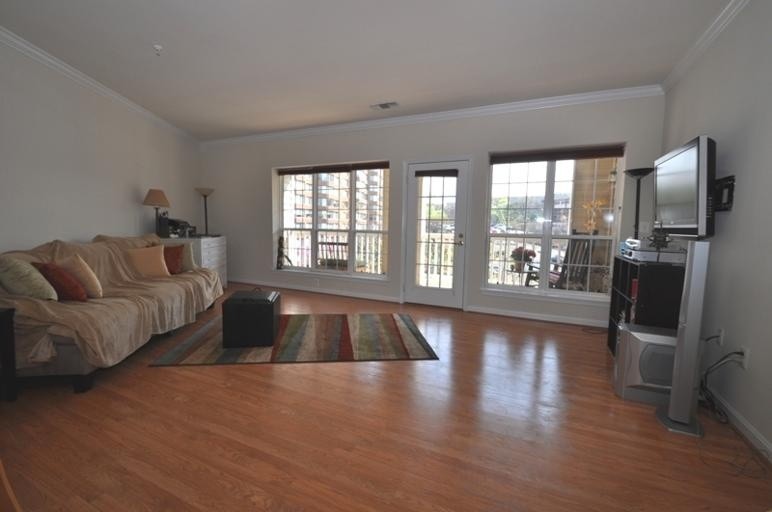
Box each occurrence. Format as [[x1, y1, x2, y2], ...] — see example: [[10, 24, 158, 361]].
[[510, 247, 536, 258]]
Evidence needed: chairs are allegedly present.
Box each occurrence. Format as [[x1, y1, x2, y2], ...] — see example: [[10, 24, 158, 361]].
[[525, 229, 598, 292]]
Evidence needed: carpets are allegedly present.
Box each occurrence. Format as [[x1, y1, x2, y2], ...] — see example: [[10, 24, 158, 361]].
[[148, 314, 439, 366]]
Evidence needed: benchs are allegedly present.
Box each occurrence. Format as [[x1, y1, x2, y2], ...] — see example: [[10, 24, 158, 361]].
[[316, 242, 365, 271]]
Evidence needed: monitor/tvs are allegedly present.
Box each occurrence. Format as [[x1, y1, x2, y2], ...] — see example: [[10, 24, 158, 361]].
[[652, 135, 716, 241]]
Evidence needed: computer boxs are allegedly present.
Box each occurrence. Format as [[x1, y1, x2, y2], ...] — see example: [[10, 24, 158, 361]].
[[614, 323, 677, 407]]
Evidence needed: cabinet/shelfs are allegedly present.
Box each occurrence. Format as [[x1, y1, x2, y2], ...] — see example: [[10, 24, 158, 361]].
[[607, 255, 686, 357], [159, 234, 227, 290]]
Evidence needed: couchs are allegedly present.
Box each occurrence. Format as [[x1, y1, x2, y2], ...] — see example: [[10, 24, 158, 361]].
[[1, 235, 224, 393]]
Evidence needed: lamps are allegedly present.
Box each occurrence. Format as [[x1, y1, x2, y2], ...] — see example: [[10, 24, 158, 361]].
[[194, 188, 221, 237], [622, 168, 654, 239], [143, 189, 170, 236]]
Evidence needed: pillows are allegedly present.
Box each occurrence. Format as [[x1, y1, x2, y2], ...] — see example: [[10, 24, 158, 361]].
[[128, 242, 198, 278], [1, 253, 103, 303]]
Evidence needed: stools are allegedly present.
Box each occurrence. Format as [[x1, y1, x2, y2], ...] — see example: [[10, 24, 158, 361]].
[[221, 290, 281, 348]]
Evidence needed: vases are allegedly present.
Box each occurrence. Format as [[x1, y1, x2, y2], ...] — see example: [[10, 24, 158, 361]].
[[515, 259, 525, 272]]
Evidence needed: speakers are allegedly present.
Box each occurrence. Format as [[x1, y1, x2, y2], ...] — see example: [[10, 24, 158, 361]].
[[667, 240, 711, 425]]
